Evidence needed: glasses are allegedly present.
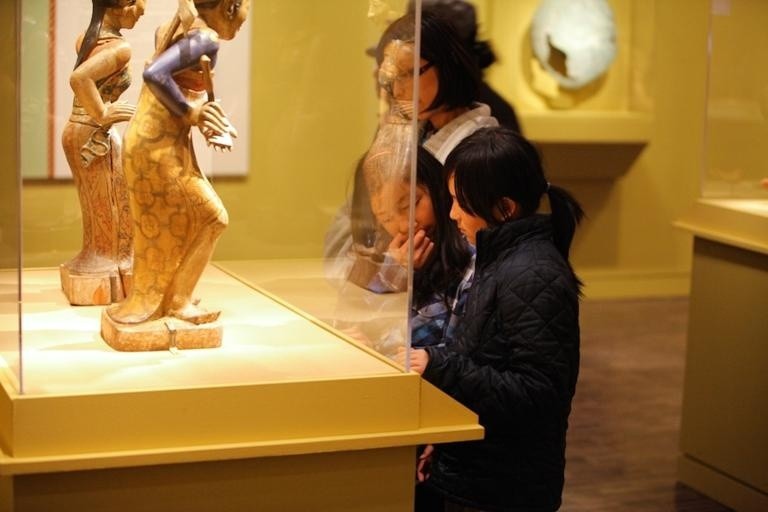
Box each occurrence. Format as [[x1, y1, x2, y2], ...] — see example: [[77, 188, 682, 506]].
[[379, 61, 439, 91]]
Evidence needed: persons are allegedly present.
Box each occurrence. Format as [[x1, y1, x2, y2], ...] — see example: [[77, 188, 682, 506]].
[[62, 1, 147, 276], [394, 127, 589, 511], [367, 0, 521, 271], [346, 141, 477, 360], [105, 0, 249, 324]]
